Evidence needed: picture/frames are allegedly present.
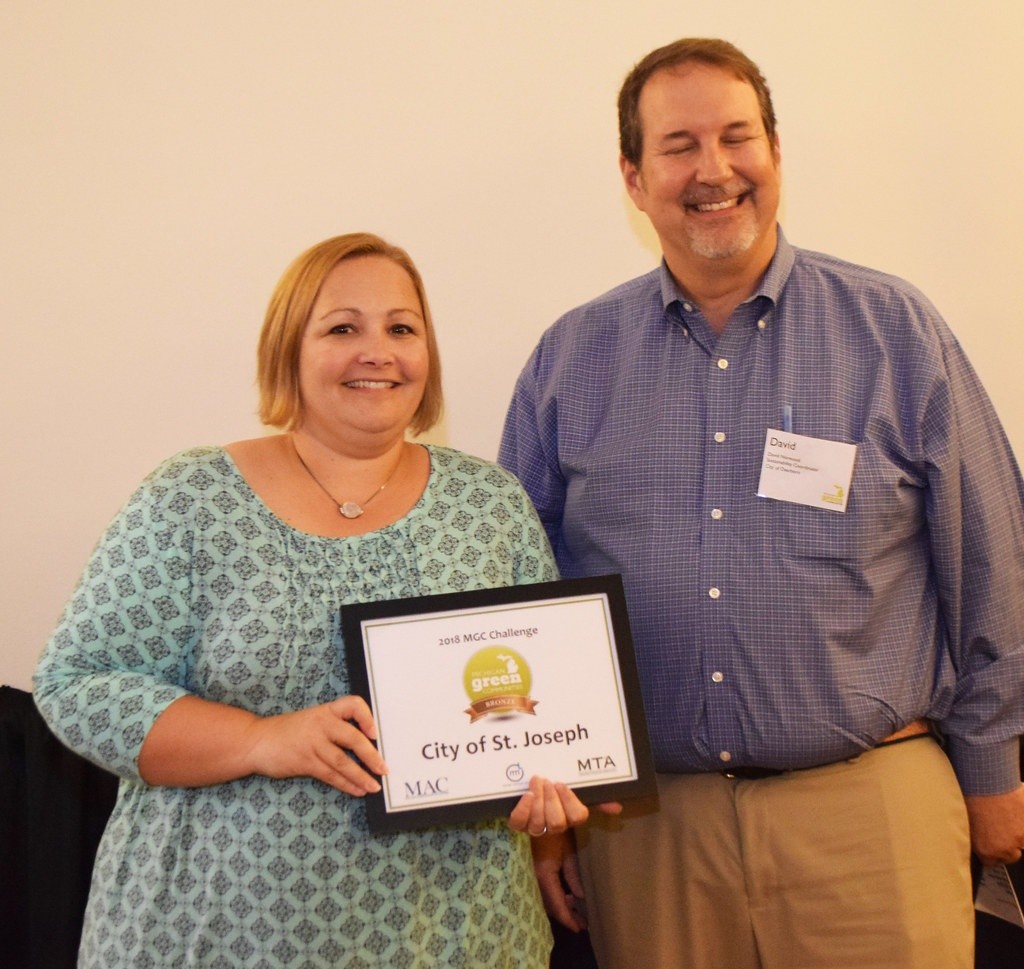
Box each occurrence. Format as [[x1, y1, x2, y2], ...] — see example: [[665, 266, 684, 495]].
[[340, 573, 660, 838]]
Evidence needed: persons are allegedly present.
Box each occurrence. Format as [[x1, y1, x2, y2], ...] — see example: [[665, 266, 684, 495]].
[[498, 38, 1024, 969], [35, 233, 623, 969]]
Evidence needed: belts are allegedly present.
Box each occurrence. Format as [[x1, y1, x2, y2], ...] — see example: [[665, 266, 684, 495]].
[[722, 721, 934, 781]]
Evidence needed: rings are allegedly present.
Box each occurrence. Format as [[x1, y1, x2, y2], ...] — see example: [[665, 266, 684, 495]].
[[527, 827, 547, 837]]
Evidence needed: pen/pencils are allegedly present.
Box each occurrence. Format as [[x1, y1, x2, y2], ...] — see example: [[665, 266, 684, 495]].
[[783, 405, 793, 434]]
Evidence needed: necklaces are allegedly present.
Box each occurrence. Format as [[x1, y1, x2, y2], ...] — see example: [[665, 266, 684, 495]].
[[290, 438, 401, 520]]
